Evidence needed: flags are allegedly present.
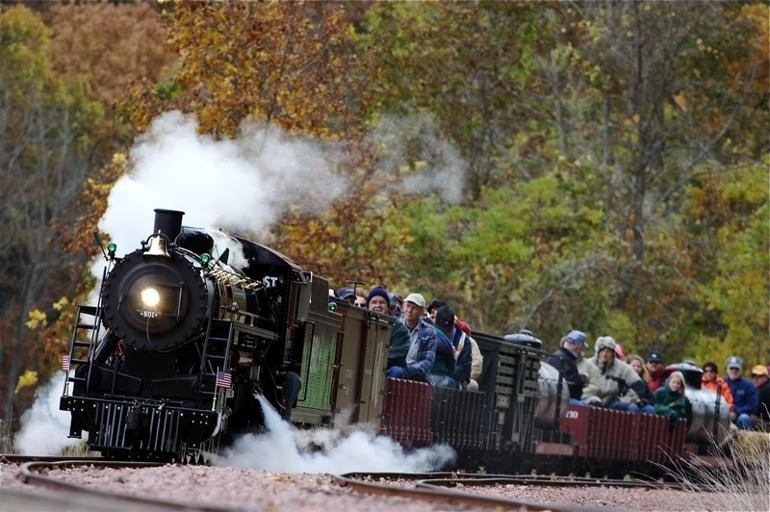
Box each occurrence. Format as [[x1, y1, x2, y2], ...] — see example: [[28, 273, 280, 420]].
[[61, 355, 69, 370], [216, 371, 232, 389]]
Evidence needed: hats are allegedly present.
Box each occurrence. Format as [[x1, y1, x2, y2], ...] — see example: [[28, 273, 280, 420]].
[[567, 330, 589, 348], [595, 335, 615, 352], [615, 343, 624, 358], [402, 293, 426, 308], [647, 352, 662, 362], [727, 356, 743, 369], [367, 286, 390, 305], [703, 362, 719, 373], [751, 364, 768, 375], [436, 306, 454, 327], [338, 287, 357, 299]]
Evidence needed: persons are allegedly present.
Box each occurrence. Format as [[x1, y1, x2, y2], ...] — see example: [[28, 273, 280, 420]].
[[340, 288, 485, 391], [701, 360, 736, 419], [549, 331, 693, 419], [723, 355, 759, 430], [751, 365, 770, 433]]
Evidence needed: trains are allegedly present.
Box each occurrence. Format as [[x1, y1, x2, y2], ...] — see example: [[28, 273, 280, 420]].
[[55, 206, 769, 488]]
